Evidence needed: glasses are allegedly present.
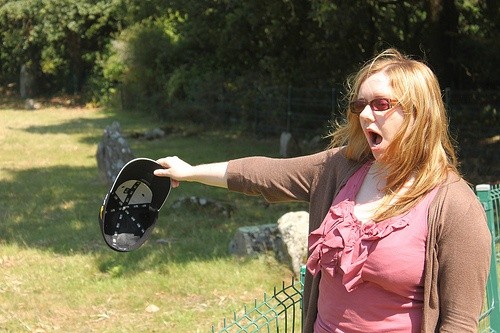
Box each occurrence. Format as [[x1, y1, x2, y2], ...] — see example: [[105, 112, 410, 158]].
[[349, 97, 404, 113]]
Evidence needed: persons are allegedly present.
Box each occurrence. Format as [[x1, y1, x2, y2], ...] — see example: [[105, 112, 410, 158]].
[[154, 47, 493, 333]]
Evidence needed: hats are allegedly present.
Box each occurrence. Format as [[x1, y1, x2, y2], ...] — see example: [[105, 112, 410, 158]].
[[99, 157, 172, 252]]
